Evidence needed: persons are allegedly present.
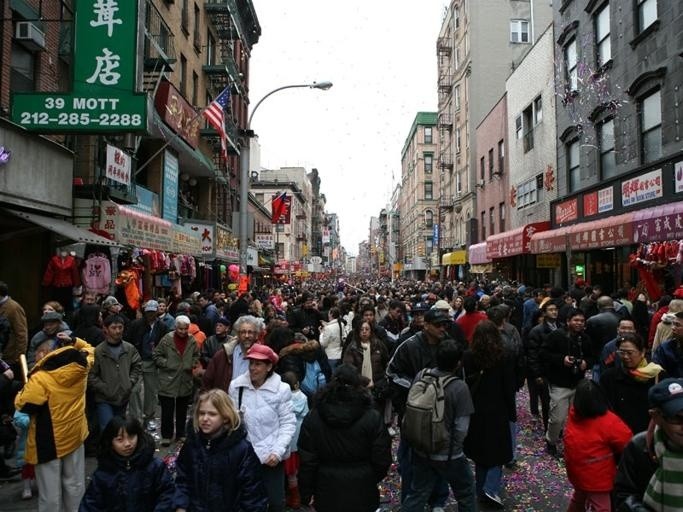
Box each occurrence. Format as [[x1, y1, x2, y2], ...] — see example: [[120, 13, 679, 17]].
[[1, 257, 682, 498], [76, 411, 173, 511], [631, 376, 683, 511], [296, 360, 392, 512], [7, 326, 96, 512], [225, 341, 295, 512], [172, 386, 272, 512], [558, 375, 633, 511], [399, 337, 480, 512]]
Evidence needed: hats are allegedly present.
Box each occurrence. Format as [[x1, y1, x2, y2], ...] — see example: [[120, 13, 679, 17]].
[[409, 299, 452, 323], [41, 295, 280, 365], [647, 377, 683, 416]]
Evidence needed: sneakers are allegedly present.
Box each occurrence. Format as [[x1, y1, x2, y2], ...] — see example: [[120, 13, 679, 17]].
[[144, 419, 156, 430], [482, 490, 504, 507], [21, 489, 32, 500], [159, 438, 170, 446]]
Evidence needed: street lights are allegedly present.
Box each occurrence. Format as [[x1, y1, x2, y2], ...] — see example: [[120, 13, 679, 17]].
[[237, 80, 332, 290]]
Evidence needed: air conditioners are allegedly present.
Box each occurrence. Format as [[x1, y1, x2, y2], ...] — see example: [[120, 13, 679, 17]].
[[16, 21, 47, 50]]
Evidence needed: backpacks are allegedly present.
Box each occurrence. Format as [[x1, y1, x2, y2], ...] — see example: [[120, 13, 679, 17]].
[[401, 368, 459, 452]]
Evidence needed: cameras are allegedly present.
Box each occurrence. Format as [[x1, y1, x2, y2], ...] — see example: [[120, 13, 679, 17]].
[[308, 325, 316, 339], [569, 356, 582, 376]]
